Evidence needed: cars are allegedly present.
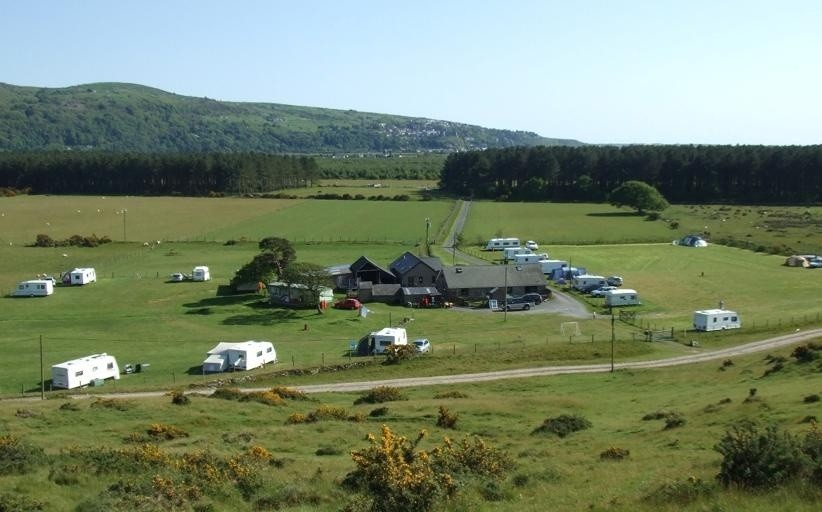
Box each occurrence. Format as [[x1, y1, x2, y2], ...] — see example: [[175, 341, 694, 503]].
[[170, 273, 183, 281], [803, 254, 822, 267], [524, 240, 536, 249], [333, 298, 361, 310], [579, 286, 618, 296], [502, 293, 543, 311], [410, 339, 430, 354], [606, 276, 622, 286]]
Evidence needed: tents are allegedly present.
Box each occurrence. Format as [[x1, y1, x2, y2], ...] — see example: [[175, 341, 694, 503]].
[[680, 234, 707, 247]]
